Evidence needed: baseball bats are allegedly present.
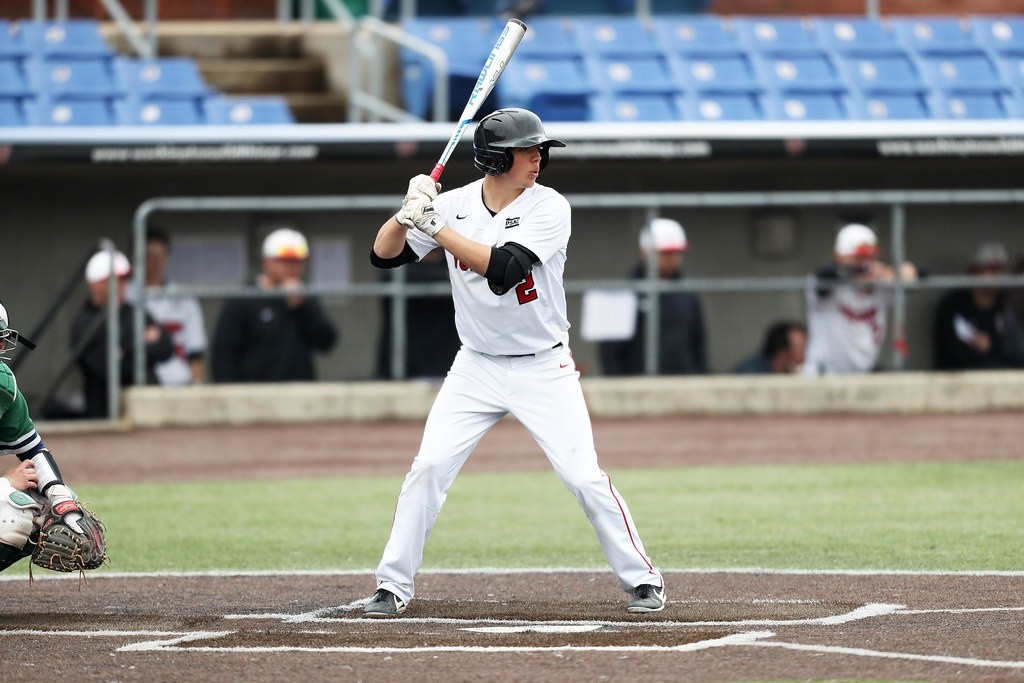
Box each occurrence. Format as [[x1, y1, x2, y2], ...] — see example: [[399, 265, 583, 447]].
[[400, 16, 528, 230]]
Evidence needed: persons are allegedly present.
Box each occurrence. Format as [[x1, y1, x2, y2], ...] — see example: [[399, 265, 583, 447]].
[[933, 243, 1024, 371], [734, 224, 926, 374], [376, 249, 461, 378], [360, 109, 667, 614], [598, 220, 706, 376], [211, 228, 340, 382], [69, 229, 207, 418], [0, 303, 107, 570]]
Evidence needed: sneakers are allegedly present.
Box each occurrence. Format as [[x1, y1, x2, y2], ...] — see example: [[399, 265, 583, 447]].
[[627, 584, 667, 612], [363, 589, 406, 616]]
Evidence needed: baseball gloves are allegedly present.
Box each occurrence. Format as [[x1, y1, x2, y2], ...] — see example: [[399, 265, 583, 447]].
[[26, 500, 112, 593]]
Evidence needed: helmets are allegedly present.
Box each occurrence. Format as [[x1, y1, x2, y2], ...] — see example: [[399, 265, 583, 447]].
[[472, 107, 567, 176], [0, 304, 36, 362]]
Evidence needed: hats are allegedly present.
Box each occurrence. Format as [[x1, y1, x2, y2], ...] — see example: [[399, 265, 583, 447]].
[[833, 224, 879, 258], [262, 228, 311, 261], [84, 249, 130, 283], [638, 218, 690, 254]]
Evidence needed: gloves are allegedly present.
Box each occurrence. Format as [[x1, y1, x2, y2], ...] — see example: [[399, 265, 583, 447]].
[[396, 174, 441, 228], [405, 193, 446, 237]]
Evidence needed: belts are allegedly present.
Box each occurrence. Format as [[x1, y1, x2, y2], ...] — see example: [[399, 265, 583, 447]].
[[509, 342, 562, 359]]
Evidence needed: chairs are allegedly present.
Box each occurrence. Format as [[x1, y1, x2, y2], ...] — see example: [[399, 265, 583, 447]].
[[403, 17, 1024, 121], [0, 19, 297, 127]]
